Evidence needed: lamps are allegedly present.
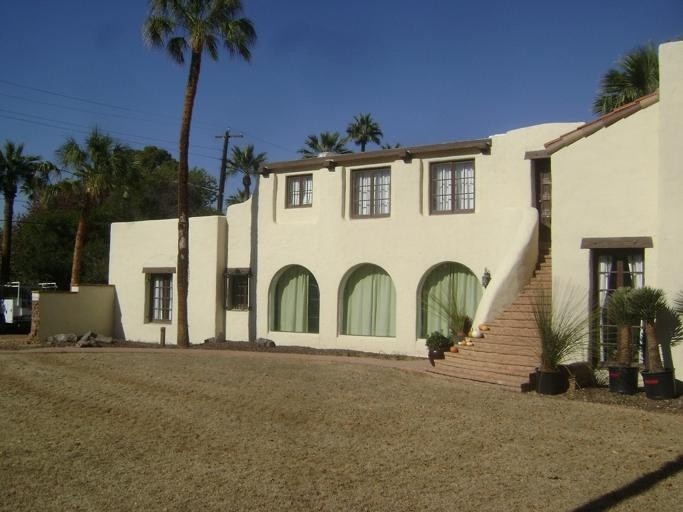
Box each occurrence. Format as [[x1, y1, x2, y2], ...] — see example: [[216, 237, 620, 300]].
[[481, 272, 491, 288]]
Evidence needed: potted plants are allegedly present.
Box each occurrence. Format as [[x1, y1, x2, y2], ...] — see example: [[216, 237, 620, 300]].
[[530, 285, 603, 395], [606, 287, 641, 395], [623, 287, 678, 399], [429, 293, 469, 344], [424, 331, 450, 366]]
